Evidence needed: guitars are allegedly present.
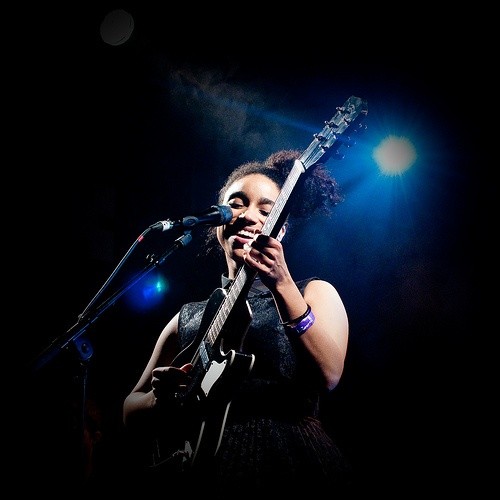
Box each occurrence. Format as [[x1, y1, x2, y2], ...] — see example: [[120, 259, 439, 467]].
[[152, 95, 369, 474]]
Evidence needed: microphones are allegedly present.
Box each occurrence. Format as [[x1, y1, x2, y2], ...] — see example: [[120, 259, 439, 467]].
[[149, 204, 234, 231]]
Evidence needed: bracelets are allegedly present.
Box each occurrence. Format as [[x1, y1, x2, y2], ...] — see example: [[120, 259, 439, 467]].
[[290, 310, 315, 337], [279, 303, 311, 325]]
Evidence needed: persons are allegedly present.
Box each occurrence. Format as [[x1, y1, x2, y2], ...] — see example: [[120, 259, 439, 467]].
[[122, 150, 349, 500]]
